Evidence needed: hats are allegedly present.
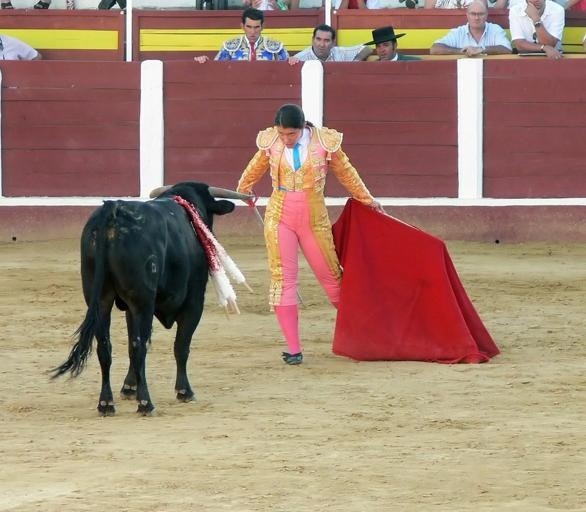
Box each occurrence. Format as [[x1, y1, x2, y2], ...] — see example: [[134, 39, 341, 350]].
[[363, 26, 406, 46]]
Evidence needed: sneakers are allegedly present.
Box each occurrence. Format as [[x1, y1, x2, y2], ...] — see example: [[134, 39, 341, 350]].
[[282, 352, 302, 365]]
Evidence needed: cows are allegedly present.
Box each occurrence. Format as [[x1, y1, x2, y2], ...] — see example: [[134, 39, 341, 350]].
[[47, 181, 256, 417]]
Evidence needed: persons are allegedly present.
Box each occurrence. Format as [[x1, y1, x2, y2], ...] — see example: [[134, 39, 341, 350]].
[[429, 1, 512, 57], [0, 35, 42, 61], [236, 104, 386, 365], [194, 8, 300, 66], [293, 24, 365, 66], [352, 26, 424, 62], [509, 0, 565, 61]]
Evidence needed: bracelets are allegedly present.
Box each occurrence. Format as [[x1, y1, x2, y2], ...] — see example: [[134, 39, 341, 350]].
[[541, 44, 545, 51], [482, 46, 486, 53], [535, 21, 543, 26]]
